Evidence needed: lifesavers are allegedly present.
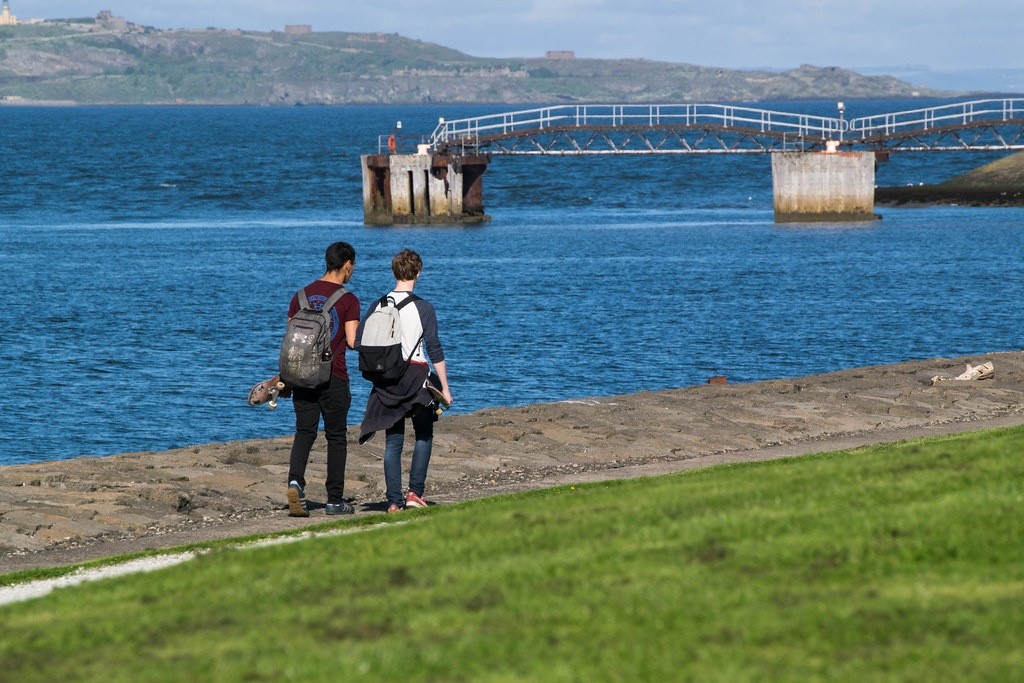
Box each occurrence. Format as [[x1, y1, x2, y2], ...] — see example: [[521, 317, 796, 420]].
[[388, 135, 397, 151]]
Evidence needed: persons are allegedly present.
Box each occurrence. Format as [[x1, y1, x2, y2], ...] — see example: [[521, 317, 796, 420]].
[[354, 248, 453, 512], [278, 241, 361, 517]]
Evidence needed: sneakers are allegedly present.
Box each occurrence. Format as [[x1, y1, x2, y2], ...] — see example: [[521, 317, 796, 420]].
[[388, 505, 403, 512], [327, 501, 354, 515], [406, 492, 428, 508], [286, 484, 310, 517]]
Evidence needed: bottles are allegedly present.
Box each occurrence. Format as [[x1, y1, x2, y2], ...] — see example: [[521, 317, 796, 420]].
[[323, 352, 331, 362]]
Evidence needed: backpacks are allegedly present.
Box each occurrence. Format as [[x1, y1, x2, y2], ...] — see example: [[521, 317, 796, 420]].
[[279, 289, 352, 389], [359, 294, 422, 385]]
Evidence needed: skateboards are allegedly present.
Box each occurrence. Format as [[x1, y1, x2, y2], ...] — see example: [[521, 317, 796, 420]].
[[426, 372, 451, 415], [247, 373, 291, 410]]
